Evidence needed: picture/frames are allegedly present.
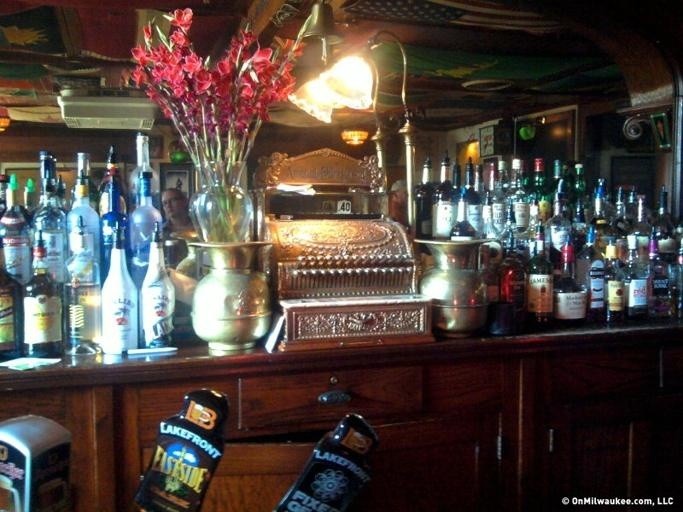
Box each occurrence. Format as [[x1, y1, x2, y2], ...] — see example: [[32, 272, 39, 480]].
[[159, 162, 193, 197], [516, 110, 575, 160]]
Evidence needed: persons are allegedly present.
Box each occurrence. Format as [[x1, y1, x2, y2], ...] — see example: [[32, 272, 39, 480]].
[[160, 188, 196, 258]]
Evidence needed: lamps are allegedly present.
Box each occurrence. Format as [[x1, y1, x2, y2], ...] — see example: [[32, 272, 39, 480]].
[[313, 27, 418, 241], [536, 116, 546, 123], [287, 47, 400, 218], [339, 129, 368, 147]]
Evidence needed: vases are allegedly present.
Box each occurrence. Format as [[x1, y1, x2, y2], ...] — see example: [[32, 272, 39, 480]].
[[414, 237, 492, 338], [187, 157, 258, 243], [188, 243, 272, 351]]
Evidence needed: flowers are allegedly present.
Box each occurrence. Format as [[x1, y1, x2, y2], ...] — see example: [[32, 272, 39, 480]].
[[131, 8, 304, 243]]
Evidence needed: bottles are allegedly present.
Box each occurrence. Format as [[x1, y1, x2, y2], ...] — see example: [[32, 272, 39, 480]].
[[416, 151, 681, 328], [0, 131, 176, 359]]
